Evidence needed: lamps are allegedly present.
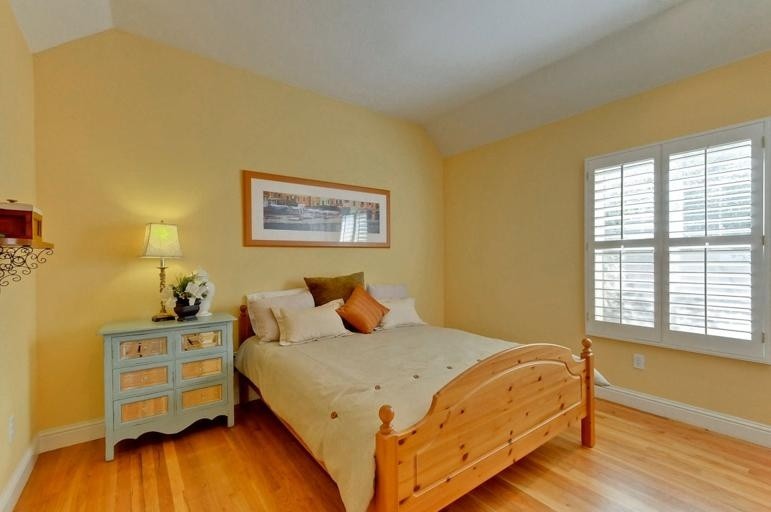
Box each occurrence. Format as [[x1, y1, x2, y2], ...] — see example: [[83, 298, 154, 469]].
[[138, 219, 185, 321]]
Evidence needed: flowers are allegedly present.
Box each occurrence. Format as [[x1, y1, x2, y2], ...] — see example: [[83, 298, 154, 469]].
[[159, 271, 210, 314]]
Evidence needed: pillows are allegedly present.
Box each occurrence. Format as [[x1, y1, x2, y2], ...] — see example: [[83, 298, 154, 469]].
[[271, 298, 355, 345], [244, 287, 317, 343], [372, 296, 428, 330], [367, 281, 410, 304], [304, 271, 366, 307], [335, 282, 392, 335]]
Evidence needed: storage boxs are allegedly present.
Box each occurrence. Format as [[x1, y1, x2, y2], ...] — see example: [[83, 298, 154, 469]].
[[0, 197, 45, 240]]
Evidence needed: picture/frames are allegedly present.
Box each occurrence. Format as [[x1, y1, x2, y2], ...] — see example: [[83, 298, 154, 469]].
[[243, 170, 391, 249]]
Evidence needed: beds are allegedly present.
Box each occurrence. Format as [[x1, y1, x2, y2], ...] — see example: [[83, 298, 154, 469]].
[[233, 305, 613, 512]]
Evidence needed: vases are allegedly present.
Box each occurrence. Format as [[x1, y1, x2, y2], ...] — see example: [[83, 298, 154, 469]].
[[172, 300, 202, 321]]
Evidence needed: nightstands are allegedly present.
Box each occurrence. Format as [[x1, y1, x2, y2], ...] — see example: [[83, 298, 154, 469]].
[[96, 311, 238, 461]]
